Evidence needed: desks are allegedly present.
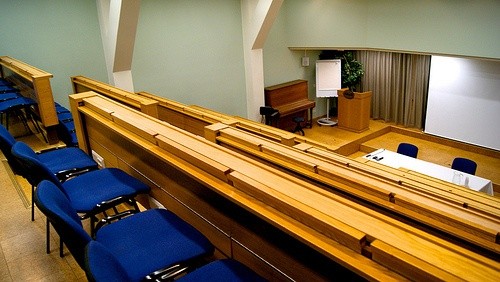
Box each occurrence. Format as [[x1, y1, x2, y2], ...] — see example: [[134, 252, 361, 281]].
[[361, 148, 495, 198]]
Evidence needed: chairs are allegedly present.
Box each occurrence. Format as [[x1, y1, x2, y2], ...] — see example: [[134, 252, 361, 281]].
[[54, 101, 81, 146], [9, 140, 150, 258], [0, 96, 48, 143], [34, 179, 215, 282], [0, 86, 16, 92], [0, 78, 9, 86], [397, 142, 418, 158], [84, 240, 271, 282], [0, 123, 98, 220], [451, 158, 478, 175], [0, 93, 40, 135]]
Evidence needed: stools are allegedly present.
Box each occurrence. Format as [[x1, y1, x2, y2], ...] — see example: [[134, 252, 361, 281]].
[[292, 116, 305, 136]]
